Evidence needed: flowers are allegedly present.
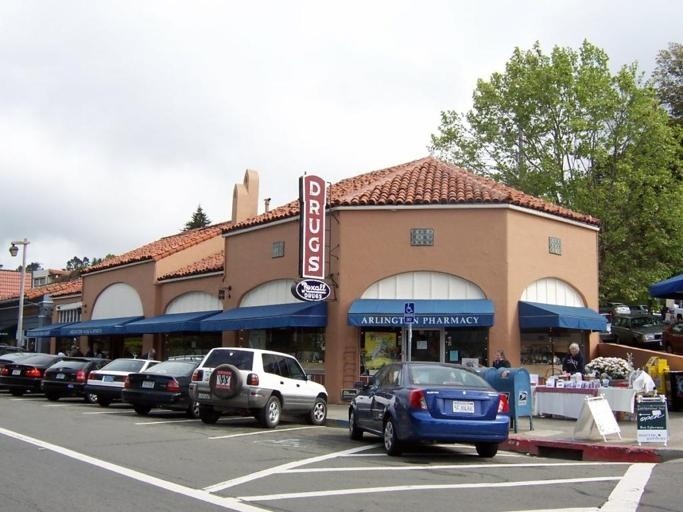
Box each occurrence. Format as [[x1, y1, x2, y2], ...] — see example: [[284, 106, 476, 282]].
[[584, 356, 631, 380]]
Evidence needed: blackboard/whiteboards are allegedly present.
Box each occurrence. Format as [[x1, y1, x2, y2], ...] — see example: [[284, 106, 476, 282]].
[[587, 398, 621, 436], [637, 402, 667, 442]]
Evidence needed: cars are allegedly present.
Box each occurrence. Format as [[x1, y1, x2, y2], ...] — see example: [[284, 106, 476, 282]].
[[349, 361, 510, 457], [600, 302, 683, 355]]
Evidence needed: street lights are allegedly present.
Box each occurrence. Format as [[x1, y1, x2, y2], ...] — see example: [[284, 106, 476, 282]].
[[9, 238, 30, 347]]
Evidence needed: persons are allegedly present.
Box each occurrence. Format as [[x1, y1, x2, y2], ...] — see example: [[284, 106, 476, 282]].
[[493, 349, 511, 368], [68, 345, 82, 358], [560, 342, 586, 377], [84, 346, 95, 357]]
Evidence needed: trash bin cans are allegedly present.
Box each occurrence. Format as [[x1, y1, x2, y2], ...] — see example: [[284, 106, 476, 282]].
[[484, 367, 533, 433]]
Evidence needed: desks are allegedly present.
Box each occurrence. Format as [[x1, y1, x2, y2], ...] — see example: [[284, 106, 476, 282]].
[[534, 377, 643, 424]]
